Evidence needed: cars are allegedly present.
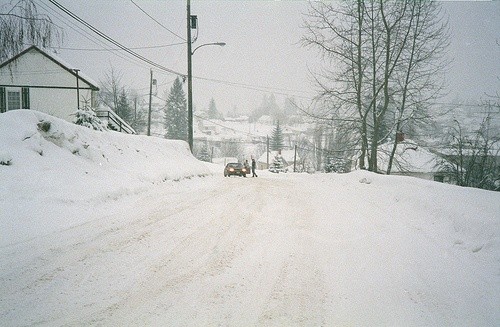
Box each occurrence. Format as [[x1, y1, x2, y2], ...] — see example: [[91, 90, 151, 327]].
[[224, 162, 247, 178]]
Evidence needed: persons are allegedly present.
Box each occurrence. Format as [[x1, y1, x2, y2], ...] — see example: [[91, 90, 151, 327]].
[[251, 158, 258, 177], [243, 159, 250, 177]]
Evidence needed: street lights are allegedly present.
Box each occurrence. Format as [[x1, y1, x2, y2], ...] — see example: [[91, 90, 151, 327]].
[[187, 42, 225, 154]]
